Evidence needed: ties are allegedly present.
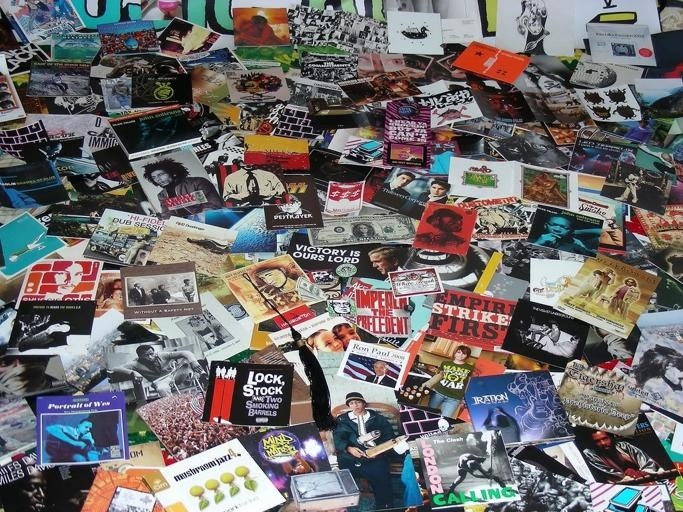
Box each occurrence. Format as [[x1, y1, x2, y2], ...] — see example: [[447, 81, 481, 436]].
[[374, 377, 379, 384], [246, 172, 259, 194]]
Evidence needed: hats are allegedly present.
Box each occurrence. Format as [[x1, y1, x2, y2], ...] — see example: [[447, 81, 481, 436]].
[[345, 392, 367, 406]]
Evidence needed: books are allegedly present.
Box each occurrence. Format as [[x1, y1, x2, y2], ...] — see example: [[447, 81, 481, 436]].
[[1, 0, 683, 512]]
